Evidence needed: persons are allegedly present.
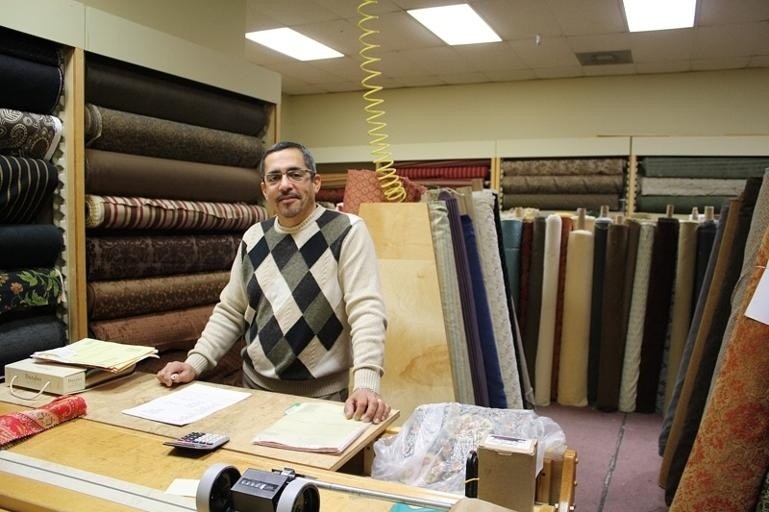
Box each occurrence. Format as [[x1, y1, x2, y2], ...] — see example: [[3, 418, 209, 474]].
[[154, 141, 393, 475]]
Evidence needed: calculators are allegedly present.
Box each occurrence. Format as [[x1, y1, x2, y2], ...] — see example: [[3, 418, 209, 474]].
[[162, 432, 230, 455]]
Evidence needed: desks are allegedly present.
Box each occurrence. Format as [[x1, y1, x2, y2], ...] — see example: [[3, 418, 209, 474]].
[[0, 352, 463, 512]]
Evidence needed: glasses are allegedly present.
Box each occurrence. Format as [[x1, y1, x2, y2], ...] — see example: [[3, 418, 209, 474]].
[[264, 166, 315, 185]]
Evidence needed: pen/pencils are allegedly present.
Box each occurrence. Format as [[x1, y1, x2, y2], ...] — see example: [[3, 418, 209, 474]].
[[170, 375, 177, 380]]
